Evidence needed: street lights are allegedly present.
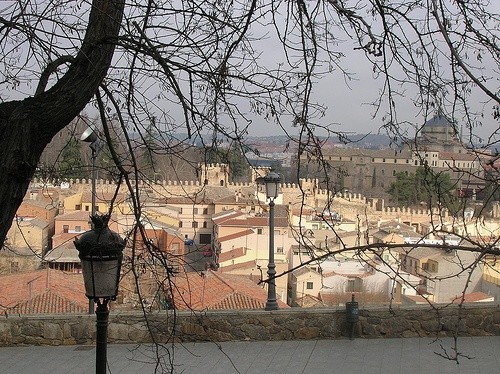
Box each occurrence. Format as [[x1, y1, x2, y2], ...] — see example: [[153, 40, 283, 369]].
[[71, 214, 126, 374], [79, 124, 98, 230], [260, 168, 283, 311]]
